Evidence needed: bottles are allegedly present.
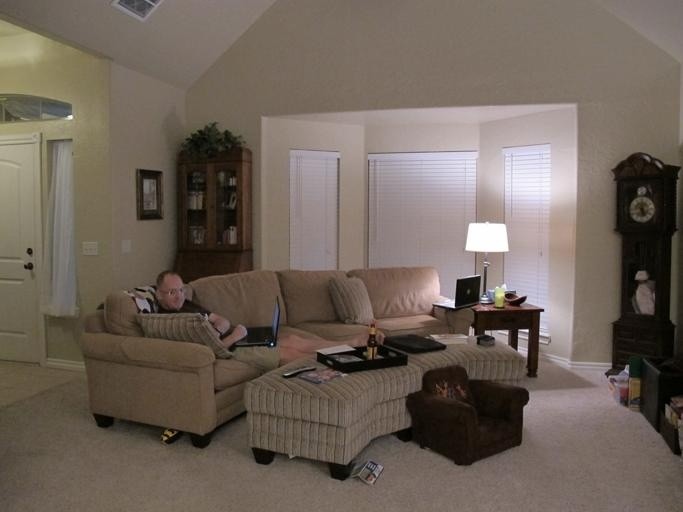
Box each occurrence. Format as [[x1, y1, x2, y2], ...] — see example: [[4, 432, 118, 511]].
[[367, 323, 378, 360], [189, 191, 205, 209]]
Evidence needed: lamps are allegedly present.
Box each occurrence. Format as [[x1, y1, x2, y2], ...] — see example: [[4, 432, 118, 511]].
[[465, 222, 510, 304]]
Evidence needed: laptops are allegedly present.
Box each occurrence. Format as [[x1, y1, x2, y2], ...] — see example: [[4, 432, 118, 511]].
[[235, 296, 280, 346], [432, 275, 481, 310]]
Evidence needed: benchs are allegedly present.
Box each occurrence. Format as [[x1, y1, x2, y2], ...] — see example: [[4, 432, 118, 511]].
[[243, 333, 526, 480]]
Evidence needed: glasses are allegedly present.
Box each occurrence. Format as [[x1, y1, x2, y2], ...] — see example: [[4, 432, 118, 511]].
[[159, 287, 186, 298]]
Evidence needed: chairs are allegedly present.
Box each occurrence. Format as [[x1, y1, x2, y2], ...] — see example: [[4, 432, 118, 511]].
[[406, 366, 529, 466]]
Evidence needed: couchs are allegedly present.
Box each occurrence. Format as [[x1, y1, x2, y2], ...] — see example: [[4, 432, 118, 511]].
[[79, 265, 476, 448]]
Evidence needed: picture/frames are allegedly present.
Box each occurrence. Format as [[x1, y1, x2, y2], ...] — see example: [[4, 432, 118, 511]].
[[136, 168, 165, 221]]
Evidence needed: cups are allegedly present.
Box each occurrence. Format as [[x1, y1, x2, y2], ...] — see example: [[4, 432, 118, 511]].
[[495, 288, 504, 308], [228, 225, 238, 245]]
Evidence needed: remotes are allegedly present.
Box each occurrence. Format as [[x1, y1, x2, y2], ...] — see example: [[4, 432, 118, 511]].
[[282, 366, 316, 378]]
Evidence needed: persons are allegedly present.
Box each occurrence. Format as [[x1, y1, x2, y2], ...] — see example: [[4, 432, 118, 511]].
[[156, 271, 300, 371]]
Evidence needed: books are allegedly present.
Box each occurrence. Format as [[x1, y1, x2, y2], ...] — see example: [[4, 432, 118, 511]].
[[350, 460, 384, 485], [430, 334, 469, 344], [300, 367, 343, 384]]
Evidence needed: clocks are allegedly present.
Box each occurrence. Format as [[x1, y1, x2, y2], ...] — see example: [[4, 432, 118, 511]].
[[612, 152, 681, 233]]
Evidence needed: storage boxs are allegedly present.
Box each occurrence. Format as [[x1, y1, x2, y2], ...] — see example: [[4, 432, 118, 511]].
[[641, 357, 682, 432], [659, 407, 680, 455]]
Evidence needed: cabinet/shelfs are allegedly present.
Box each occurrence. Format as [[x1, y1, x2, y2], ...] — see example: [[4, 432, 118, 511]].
[[177, 147, 253, 285]]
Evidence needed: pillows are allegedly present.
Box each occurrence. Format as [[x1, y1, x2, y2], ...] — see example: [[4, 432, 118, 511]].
[[136, 311, 235, 359], [328, 277, 375, 325]]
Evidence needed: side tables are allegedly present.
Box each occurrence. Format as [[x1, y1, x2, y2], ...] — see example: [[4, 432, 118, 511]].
[[471, 298, 544, 377]]
